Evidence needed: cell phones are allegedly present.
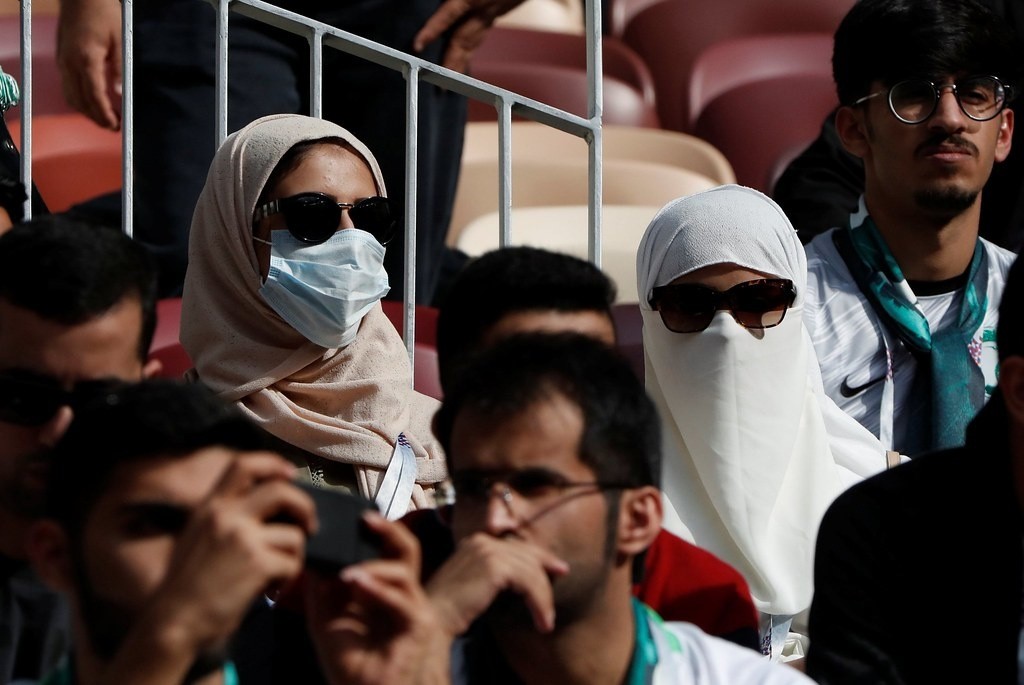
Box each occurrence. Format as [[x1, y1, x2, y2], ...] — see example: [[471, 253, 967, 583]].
[[266, 482, 385, 570]]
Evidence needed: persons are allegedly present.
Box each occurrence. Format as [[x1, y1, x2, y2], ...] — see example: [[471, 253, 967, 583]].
[[772, 0, 1024, 460], [57, 0, 527, 307], [804, 255, 1024, 685], [1, 220, 818, 685], [178, 113, 456, 521], [637, 183, 911, 668]]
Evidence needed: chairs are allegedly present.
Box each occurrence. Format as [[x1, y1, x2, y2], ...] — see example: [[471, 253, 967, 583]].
[[1, 0, 854, 398]]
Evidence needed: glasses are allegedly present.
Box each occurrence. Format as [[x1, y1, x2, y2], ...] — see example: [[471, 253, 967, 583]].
[[646, 278, 795, 335], [254, 191, 394, 247], [849, 73, 1009, 124], [0, 368, 133, 427], [431, 461, 608, 525]]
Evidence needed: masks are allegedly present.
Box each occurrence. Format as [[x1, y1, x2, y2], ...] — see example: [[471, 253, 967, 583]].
[[252, 229, 391, 349]]
